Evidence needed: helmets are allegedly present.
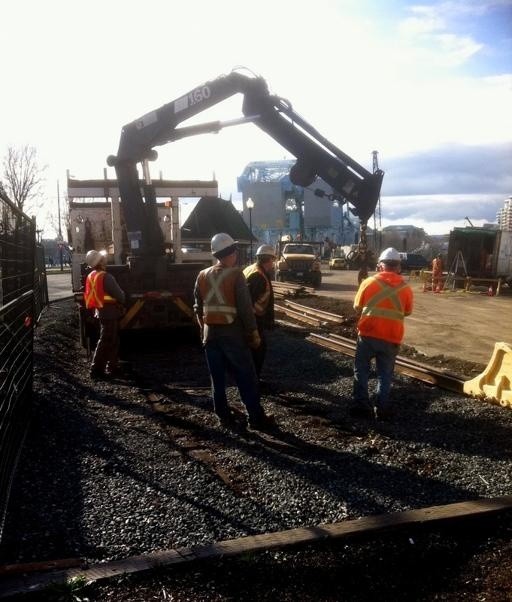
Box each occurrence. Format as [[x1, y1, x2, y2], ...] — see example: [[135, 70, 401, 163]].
[[255, 245, 276, 257], [211, 233, 238, 254], [85, 250, 104, 268], [378, 247, 403, 263]]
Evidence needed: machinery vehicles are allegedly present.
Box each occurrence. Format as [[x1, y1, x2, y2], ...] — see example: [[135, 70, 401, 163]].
[[72, 63, 385, 362]]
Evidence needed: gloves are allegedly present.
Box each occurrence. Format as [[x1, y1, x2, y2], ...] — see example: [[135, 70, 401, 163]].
[[116, 305, 127, 315], [247, 328, 261, 349]]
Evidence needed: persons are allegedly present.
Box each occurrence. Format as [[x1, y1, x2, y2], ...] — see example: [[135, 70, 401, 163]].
[[193, 232, 275, 430], [350, 246, 415, 424], [240, 244, 282, 388], [83, 249, 128, 381], [431, 254, 444, 294]]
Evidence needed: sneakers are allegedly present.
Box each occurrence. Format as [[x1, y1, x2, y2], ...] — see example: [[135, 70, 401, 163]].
[[219, 410, 235, 427], [350, 406, 385, 419], [91, 366, 123, 379], [248, 413, 274, 427]]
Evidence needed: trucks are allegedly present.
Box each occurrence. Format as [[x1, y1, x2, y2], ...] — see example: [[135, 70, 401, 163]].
[[274, 240, 325, 287], [329, 248, 347, 270], [376, 252, 425, 271]]
